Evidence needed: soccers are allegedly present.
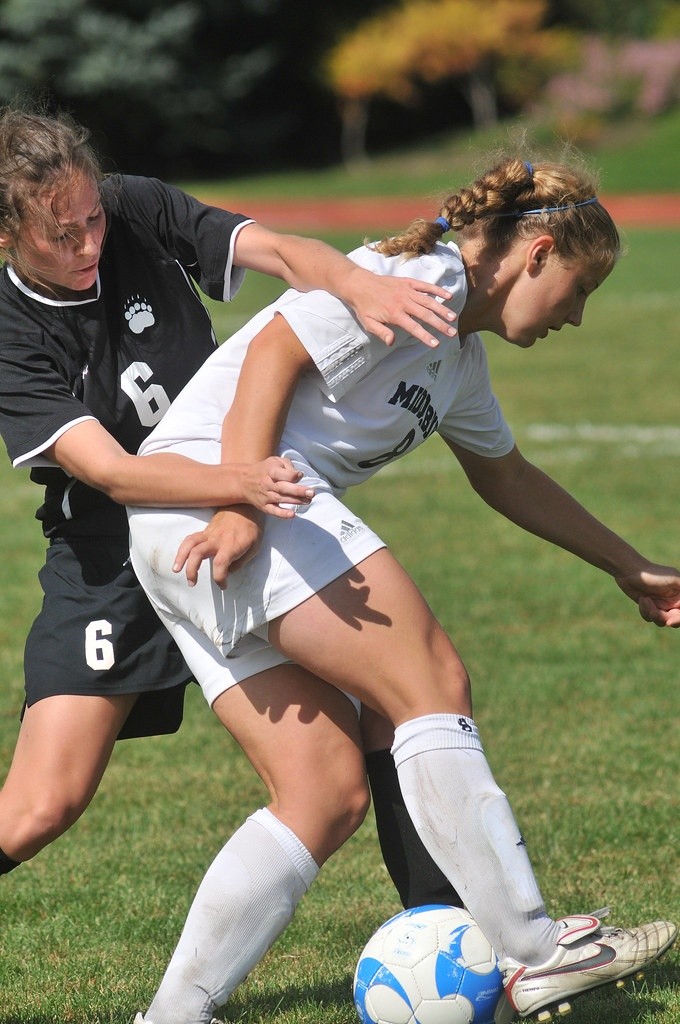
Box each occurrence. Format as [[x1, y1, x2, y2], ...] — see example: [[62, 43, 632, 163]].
[[353, 905, 516, 1024]]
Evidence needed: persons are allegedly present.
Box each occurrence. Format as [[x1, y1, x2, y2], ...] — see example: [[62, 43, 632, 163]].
[[0, 108, 620, 1024], [125, 160, 680, 1024]]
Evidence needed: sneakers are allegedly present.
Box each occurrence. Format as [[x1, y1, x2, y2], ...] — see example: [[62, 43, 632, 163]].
[[496, 916, 679, 1021]]
